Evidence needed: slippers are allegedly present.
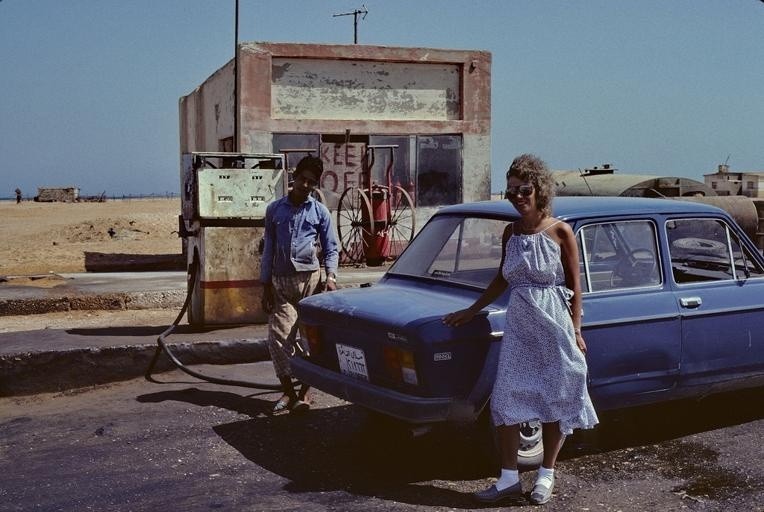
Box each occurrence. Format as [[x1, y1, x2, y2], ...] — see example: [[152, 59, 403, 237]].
[[272, 400, 310, 414]]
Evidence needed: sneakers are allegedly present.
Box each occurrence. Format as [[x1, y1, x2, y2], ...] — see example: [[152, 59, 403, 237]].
[[475, 483, 522, 503], [530, 479, 555, 505]]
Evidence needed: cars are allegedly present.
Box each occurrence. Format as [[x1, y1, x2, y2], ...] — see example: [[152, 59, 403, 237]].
[[287, 195, 764, 426]]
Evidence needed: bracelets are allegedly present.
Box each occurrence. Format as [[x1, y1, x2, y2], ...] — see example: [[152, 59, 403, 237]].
[[327, 276, 337, 284], [574, 327, 581, 334]]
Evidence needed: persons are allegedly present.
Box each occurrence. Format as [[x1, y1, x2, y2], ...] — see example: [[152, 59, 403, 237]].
[[437, 151, 603, 506], [256, 155, 340, 415], [14, 188, 23, 205]]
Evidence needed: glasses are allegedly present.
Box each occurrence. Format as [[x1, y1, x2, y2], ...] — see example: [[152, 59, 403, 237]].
[[506, 185, 532, 197]]
